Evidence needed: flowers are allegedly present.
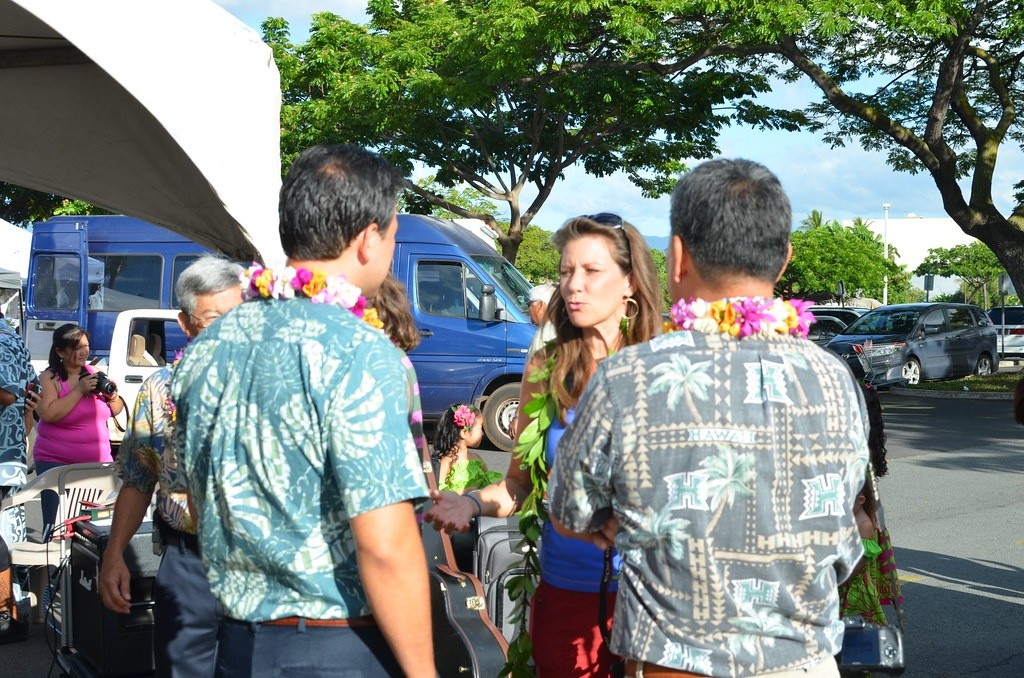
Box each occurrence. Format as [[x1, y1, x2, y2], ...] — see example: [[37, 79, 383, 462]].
[[659, 297, 818, 339], [240, 262, 386, 329], [451, 404, 476, 431]]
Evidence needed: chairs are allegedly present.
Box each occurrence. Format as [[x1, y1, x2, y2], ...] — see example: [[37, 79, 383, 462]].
[[128, 334, 151, 366], [149, 334, 165, 366], [0, 463, 123, 624]]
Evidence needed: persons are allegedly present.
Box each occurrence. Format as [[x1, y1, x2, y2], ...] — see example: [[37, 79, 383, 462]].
[[547, 158, 870, 678], [425, 215, 663, 678], [101, 258, 247, 678], [32, 323, 123, 544], [433, 403, 502, 492], [365, 270, 423, 538], [510, 284, 556, 439], [171, 146, 437, 678], [0, 312, 44, 645], [838, 381, 904, 625]]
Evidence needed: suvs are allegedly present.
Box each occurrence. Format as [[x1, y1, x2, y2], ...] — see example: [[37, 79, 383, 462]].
[[987, 304, 1024, 360], [828, 303, 1000, 389]]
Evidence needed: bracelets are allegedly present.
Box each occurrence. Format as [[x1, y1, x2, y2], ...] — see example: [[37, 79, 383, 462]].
[[461, 494, 481, 516]]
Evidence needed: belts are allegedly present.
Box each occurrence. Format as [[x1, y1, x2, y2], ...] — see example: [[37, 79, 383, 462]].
[[256, 614, 376, 627]]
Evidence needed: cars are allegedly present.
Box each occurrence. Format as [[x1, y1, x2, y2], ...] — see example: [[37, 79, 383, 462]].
[[803, 307, 881, 351]]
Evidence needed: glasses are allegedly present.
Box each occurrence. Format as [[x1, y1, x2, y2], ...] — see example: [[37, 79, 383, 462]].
[[577, 212, 632, 270], [189, 312, 212, 330]]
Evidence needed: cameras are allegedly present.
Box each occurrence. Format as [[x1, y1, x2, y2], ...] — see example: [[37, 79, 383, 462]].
[[831, 614, 906, 678], [23, 383, 44, 411], [79, 370, 116, 394]]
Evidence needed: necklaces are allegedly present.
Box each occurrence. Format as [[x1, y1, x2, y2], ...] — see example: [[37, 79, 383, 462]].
[[663, 296, 817, 338], [239, 262, 384, 329]]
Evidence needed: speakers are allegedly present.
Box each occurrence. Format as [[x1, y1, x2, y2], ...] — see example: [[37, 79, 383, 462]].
[[54, 518, 177, 678]]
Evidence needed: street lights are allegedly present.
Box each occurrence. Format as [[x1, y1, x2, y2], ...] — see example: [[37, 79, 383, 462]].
[[882, 202, 890, 307]]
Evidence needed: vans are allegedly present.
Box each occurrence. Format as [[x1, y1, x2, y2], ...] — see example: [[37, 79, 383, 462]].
[[19, 212, 542, 450]]
